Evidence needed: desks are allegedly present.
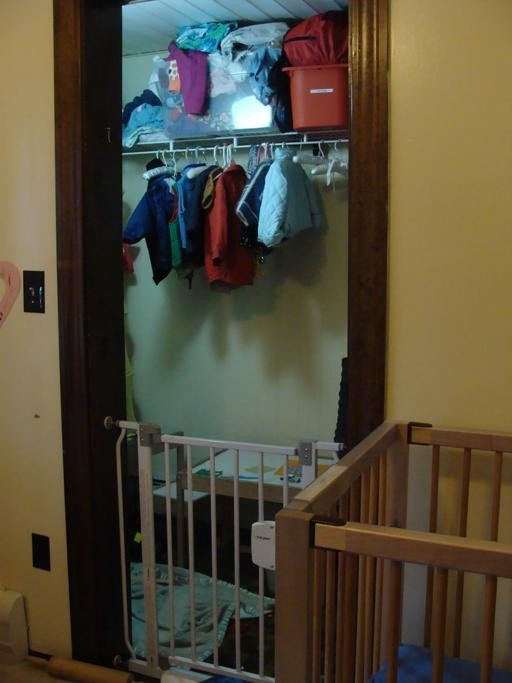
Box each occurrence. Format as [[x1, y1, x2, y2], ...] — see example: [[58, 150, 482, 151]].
[[177, 448, 337, 555]]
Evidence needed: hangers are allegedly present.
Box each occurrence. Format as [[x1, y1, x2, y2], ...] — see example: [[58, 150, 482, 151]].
[[142, 139, 348, 186]]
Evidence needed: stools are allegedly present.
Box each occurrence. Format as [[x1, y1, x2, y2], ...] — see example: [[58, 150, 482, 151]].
[[152, 479, 210, 519]]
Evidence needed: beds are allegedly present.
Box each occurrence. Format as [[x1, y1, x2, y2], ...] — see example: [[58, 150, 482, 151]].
[[275, 422, 512, 683]]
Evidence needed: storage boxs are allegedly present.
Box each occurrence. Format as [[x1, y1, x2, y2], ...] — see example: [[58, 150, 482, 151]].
[[282, 63, 349, 140]]
